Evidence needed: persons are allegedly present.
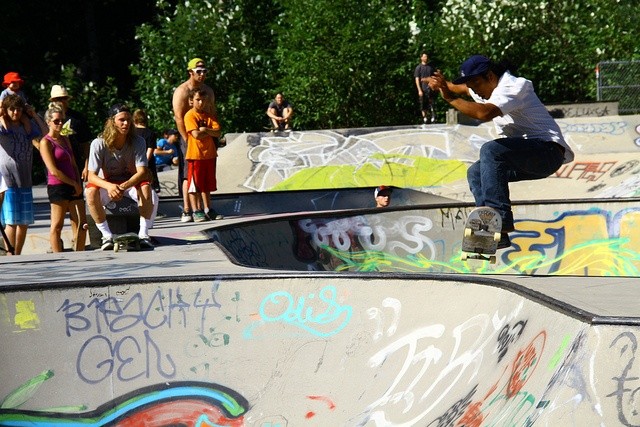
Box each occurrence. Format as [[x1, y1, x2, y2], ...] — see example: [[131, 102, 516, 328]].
[[39, 101, 87, 252], [173, 58, 224, 223], [183, 89, 223, 222], [86, 103, 160, 252], [154, 129, 179, 172], [0, 96, 49, 256], [130, 107, 159, 229], [414, 53, 438, 123], [421, 55, 574, 249], [0, 72, 33, 136], [48, 84, 90, 244], [267, 94, 294, 130], [374, 185, 393, 207]]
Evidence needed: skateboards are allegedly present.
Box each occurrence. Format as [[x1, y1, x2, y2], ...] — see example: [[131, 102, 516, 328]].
[[460, 206, 502, 264], [264, 127, 291, 133], [113, 233, 141, 252]]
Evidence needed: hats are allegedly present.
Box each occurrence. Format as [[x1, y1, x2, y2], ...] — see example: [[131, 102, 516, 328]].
[[2, 71, 24, 86], [167, 129, 179, 135], [48, 84, 73, 101], [374, 185, 393, 198], [187, 57, 205, 70], [451, 54, 492, 86], [110, 104, 131, 115]]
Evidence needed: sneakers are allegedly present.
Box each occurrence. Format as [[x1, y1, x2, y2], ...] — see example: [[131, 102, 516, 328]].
[[137, 235, 155, 251], [496, 233, 511, 250], [101, 234, 114, 251], [192, 209, 207, 223], [499, 219, 514, 233], [179, 210, 193, 223], [204, 209, 224, 220]]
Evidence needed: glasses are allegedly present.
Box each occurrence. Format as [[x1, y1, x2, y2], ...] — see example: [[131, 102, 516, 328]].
[[7, 105, 24, 112], [51, 119, 66, 126], [192, 68, 207, 74]]
